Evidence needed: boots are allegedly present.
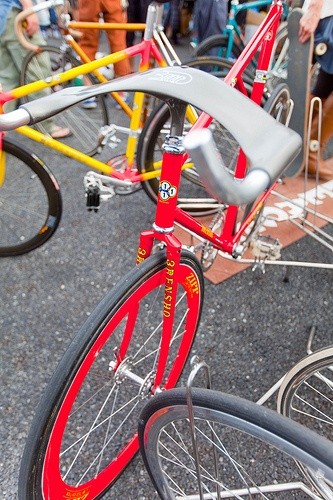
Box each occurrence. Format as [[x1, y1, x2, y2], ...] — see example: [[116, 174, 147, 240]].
[[307, 92, 333, 181]]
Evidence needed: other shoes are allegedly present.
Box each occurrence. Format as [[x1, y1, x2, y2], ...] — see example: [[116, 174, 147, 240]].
[[46, 125, 73, 139], [113, 96, 130, 109], [82, 96, 97, 109]]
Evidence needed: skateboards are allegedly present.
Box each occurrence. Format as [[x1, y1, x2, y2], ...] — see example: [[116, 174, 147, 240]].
[[279, 8, 314, 179]]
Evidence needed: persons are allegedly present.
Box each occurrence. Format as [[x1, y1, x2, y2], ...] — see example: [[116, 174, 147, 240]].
[[0, 0, 71, 138], [30, 0, 333, 180]]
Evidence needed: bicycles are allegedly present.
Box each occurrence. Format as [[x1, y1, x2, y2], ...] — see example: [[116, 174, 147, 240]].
[[0, 3, 333, 500], [248, 327, 333, 436], [136, 363, 333, 500], [0, 0, 332, 258]]
